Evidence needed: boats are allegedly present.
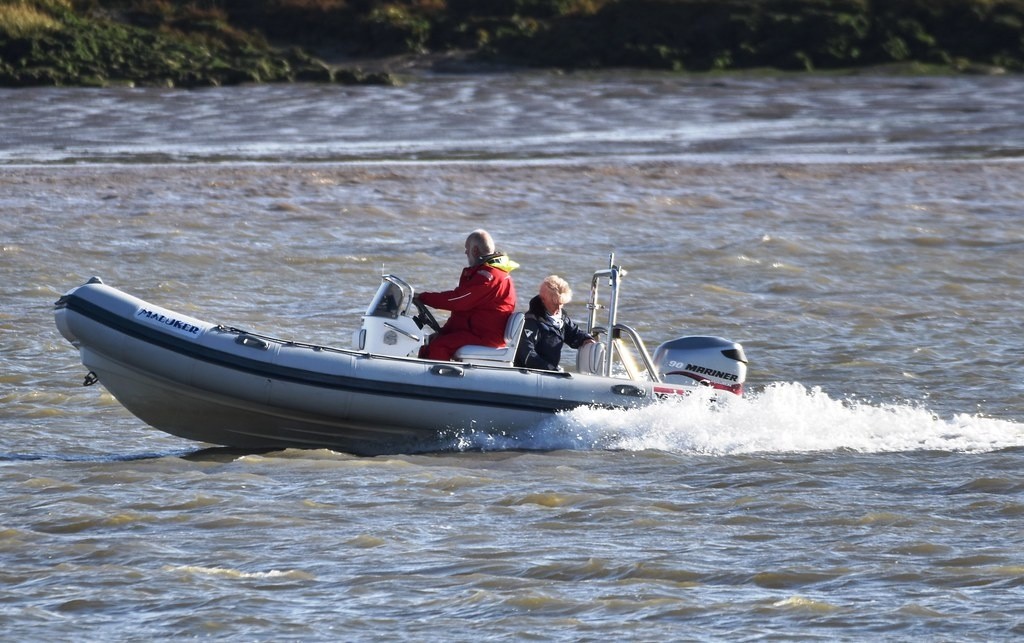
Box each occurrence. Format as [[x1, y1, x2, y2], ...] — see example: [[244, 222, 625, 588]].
[[53, 275, 747, 456]]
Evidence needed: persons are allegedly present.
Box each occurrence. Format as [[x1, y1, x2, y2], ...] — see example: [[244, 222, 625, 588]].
[[415, 229, 516, 361], [513, 275, 595, 371]]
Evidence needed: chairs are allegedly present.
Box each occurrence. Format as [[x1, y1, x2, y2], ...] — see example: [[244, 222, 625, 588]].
[[458, 313, 527, 363]]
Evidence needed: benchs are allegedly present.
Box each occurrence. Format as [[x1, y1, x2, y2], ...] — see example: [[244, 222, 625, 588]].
[[567, 342, 606, 376]]
